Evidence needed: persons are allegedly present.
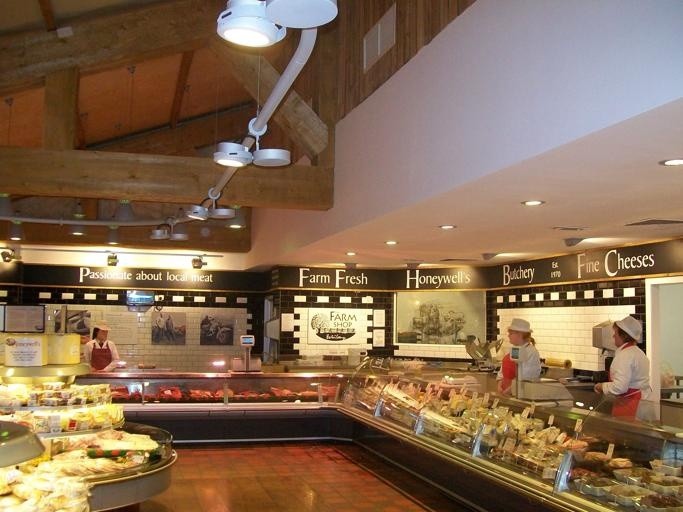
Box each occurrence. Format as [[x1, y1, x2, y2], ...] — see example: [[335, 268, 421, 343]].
[[165, 314, 178, 342], [155, 313, 165, 341], [495, 319, 542, 395], [593, 316, 657, 423], [83, 318, 119, 371]]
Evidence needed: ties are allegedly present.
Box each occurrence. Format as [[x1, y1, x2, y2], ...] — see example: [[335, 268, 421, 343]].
[[99, 342, 104, 348]]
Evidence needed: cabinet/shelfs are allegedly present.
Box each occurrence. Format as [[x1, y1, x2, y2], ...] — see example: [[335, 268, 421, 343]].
[[74, 371, 398, 450], [339, 358, 682, 512]]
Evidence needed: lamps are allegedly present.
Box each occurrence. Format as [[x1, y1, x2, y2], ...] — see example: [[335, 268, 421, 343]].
[[149, 187, 237, 243], [211, 1, 340, 169]]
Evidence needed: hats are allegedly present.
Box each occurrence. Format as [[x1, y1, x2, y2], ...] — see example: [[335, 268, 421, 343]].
[[94, 320, 111, 331], [615, 316, 643, 343], [508, 318, 531, 332]]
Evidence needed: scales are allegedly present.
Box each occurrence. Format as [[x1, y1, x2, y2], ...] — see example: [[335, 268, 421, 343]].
[[510, 346, 574, 400], [231, 335, 261, 372]]
